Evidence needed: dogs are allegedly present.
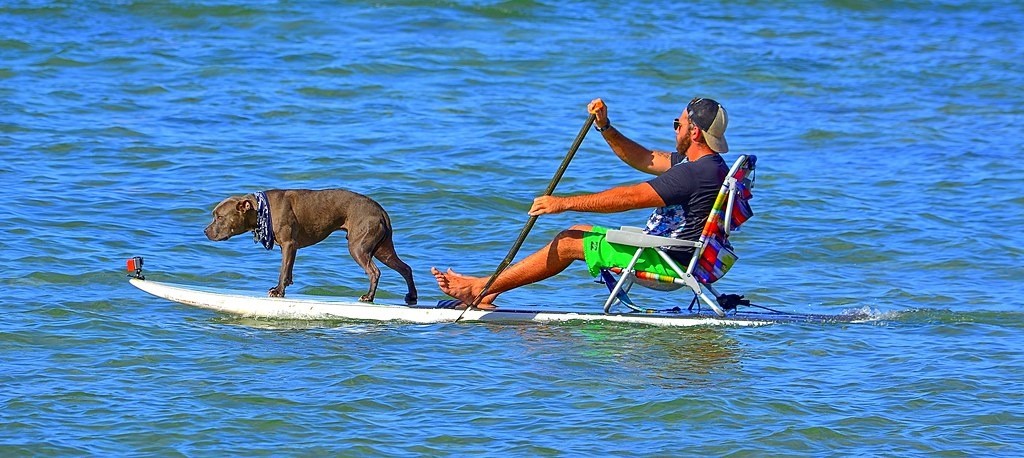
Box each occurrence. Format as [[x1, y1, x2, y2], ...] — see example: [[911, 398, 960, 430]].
[[203, 189, 418, 305]]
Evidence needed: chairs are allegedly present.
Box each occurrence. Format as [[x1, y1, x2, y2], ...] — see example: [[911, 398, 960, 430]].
[[604, 154, 757, 317]]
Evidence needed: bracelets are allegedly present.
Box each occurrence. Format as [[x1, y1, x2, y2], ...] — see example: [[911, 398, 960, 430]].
[[595, 118, 610, 132]]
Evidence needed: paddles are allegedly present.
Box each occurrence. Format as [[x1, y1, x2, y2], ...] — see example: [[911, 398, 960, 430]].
[[448, 114, 596, 322]]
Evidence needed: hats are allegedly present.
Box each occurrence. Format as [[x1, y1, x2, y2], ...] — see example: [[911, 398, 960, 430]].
[[688, 98, 729, 152]]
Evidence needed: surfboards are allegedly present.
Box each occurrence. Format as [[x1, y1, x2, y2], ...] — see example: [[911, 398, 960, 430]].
[[128, 276, 776, 327]]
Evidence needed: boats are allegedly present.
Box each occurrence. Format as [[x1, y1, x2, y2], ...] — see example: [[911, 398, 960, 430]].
[[130, 277, 881, 327]]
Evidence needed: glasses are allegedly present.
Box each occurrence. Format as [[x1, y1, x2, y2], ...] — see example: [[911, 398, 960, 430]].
[[673, 119, 692, 130]]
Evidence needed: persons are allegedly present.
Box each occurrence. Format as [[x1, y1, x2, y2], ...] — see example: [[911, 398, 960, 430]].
[[431, 97, 729, 309]]
